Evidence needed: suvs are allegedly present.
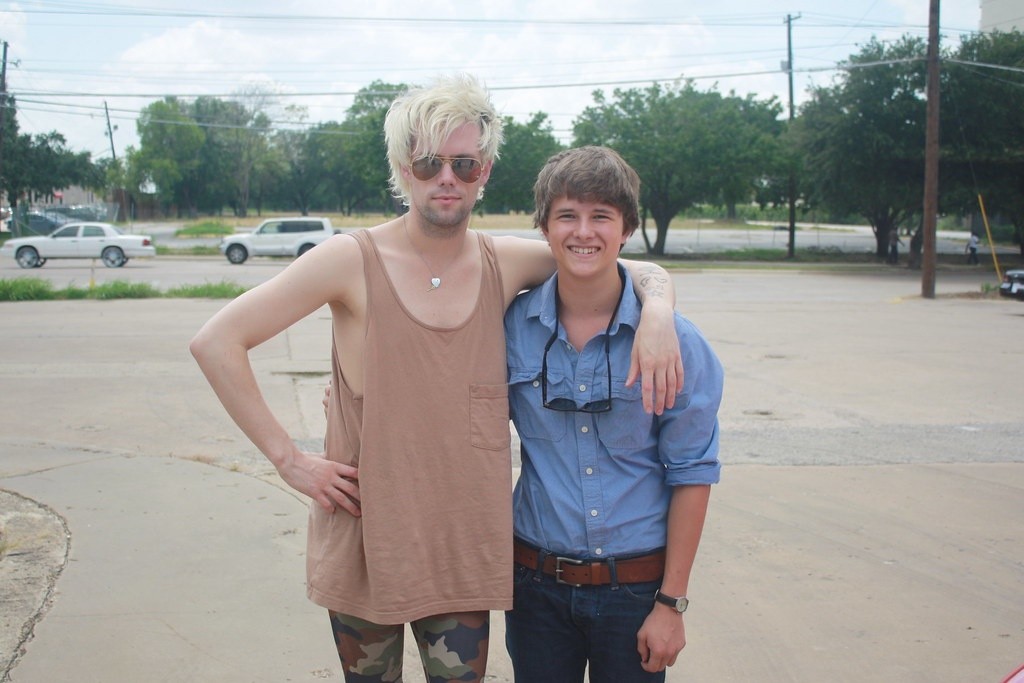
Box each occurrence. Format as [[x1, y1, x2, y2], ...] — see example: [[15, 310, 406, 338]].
[[219, 217, 333, 265]]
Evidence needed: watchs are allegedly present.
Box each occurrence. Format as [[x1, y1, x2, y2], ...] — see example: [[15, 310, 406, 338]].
[[654, 589, 689, 615]]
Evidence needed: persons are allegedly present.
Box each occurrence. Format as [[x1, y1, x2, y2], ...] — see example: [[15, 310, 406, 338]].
[[189, 71, 685, 683], [965, 231, 983, 266], [320, 145, 723, 683], [887, 224, 905, 264]]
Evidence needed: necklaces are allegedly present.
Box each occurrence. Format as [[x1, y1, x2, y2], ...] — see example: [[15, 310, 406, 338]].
[[403, 213, 467, 287]]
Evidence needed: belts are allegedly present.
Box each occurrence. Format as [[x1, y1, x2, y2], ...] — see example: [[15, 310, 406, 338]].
[[512, 540, 668, 586]]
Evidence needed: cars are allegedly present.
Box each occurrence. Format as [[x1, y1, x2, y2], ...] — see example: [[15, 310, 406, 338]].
[[6, 213, 78, 235], [44, 205, 108, 225], [1, 221, 157, 269], [999, 269, 1023, 303]]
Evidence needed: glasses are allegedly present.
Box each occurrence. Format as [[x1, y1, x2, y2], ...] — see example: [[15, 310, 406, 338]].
[[542, 347, 614, 413], [409, 153, 491, 183]]
